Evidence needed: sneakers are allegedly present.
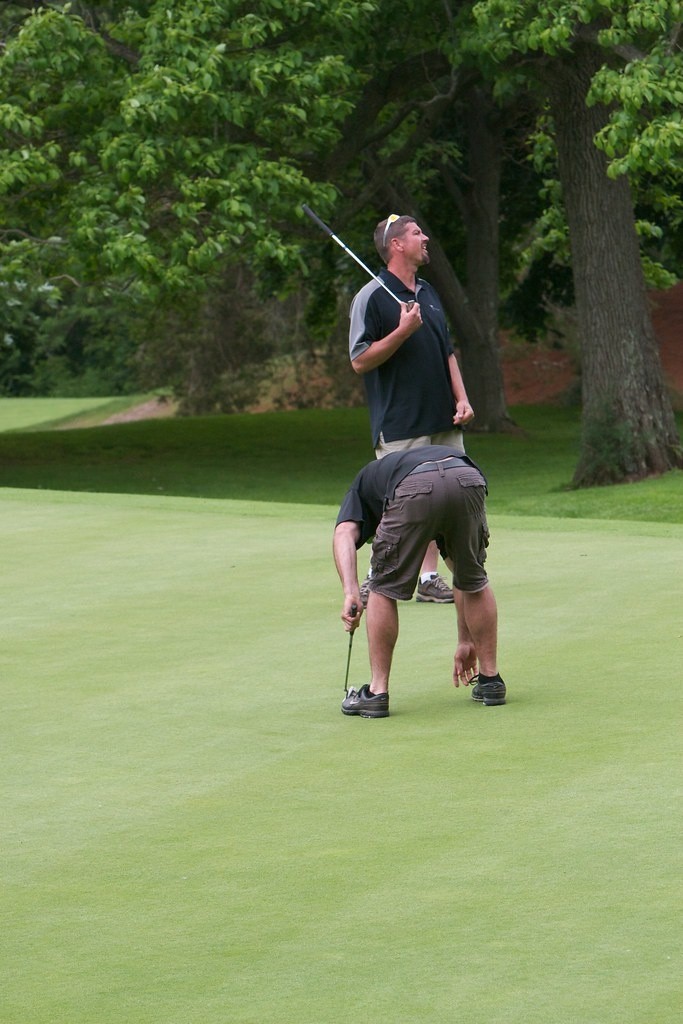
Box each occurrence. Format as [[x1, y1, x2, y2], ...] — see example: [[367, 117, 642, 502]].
[[341, 684, 389, 717], [360, 575, 370, 608], [469, 674, 506, 705], [416, 574, 454, 603]]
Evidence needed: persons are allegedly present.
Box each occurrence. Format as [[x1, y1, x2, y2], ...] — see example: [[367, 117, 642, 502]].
[[331, 444, 508, 719], [349, 215, 475, 602]]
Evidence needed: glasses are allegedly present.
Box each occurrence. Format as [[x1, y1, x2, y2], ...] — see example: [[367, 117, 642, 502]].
[[383, 214, 400, 247]]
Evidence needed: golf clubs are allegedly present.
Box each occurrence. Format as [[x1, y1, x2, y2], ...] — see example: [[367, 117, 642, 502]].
[[342, 603, 358, 707], [301, 203, 415, 313]]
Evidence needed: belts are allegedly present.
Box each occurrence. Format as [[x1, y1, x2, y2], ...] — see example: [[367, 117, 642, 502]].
[[407, 458, 472, 474]]
